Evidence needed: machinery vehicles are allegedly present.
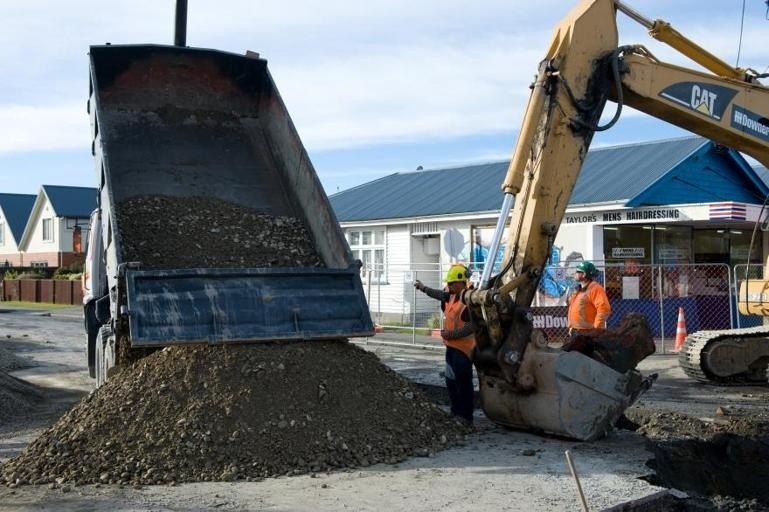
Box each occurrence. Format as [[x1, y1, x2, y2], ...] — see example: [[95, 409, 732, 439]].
[[471, 1, 769, 445]]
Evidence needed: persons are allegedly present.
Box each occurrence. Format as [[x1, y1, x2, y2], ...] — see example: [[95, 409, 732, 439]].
[[439, 262, 473, 313], [412, 266, 490, 427], [568, 261, 612, 337]]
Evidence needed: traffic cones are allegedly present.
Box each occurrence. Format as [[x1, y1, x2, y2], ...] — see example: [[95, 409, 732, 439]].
[[669, 306, 688, 354]]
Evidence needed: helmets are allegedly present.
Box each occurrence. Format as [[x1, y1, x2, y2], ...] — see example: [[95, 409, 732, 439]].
[[446, 264, 467, 282], [576, 261, 596, 278]]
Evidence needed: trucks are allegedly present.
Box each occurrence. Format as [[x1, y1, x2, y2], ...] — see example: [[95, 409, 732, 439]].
[[85, 39, 375, 387]]
